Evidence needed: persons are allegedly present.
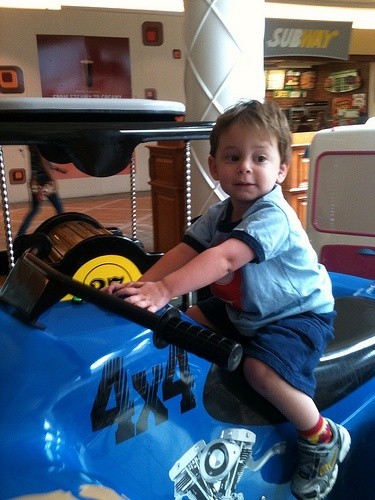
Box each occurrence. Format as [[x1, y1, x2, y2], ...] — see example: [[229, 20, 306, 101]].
[[296, 107, 369, 133], [17, 144, 69, 235], [98, 98, 352, 500]]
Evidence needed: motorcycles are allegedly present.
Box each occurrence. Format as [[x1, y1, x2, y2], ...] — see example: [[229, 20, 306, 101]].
[[1, 231, 375, 500]]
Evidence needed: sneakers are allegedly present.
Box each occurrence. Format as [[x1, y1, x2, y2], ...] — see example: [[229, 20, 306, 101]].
[[290, 418, 352, 500]]
